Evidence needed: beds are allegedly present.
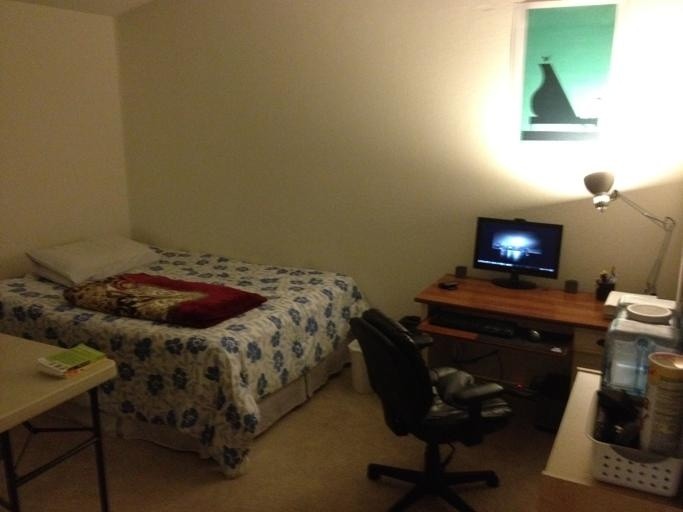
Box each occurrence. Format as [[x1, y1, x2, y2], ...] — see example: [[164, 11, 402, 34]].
[[1, 243, 370, 481]]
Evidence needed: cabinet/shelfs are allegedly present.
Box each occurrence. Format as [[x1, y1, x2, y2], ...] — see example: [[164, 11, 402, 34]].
[[537, 367, 683, 511]]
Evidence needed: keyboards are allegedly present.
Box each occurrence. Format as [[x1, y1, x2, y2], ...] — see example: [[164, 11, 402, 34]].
[[429, 311, 518, 338]]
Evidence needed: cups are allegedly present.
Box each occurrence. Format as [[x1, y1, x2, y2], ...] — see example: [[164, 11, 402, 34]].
[[455, 266, 467, 279], [564, 279, 578, 293], [595, 278, 615, 301]]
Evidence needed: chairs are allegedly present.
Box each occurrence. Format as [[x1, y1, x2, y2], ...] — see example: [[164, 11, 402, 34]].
[[348, 306, 516, 511]]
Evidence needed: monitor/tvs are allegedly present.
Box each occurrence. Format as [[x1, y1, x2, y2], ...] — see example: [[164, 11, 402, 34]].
[[473, 217, 563, 290]]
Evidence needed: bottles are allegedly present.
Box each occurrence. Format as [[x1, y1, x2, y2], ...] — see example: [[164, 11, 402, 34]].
[[639, 351, 683, 459]]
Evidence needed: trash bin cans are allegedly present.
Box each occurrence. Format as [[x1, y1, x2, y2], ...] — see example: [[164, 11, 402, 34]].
[[532, 372, 570, 430]]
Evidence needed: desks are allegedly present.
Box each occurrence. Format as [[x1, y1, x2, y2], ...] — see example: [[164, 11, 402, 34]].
[[0, 333, 118, 510], [412, 271, 614, 426]]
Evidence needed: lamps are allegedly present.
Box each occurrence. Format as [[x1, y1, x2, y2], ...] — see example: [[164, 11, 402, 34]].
[[583, 171, 676, 298]]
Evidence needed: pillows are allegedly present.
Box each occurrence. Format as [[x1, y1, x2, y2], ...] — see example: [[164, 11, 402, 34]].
[[29, 248, 160, 290], [25, 233, 151, 284]]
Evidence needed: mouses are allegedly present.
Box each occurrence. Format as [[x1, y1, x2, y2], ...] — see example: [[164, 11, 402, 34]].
[[528, 327, 545, 340]]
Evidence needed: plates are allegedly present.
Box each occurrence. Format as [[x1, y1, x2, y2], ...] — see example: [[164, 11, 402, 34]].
[[626, 302, 673, 324]]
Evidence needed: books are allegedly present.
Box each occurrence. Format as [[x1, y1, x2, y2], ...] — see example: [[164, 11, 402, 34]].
[[37, 343, 108, 379]]
